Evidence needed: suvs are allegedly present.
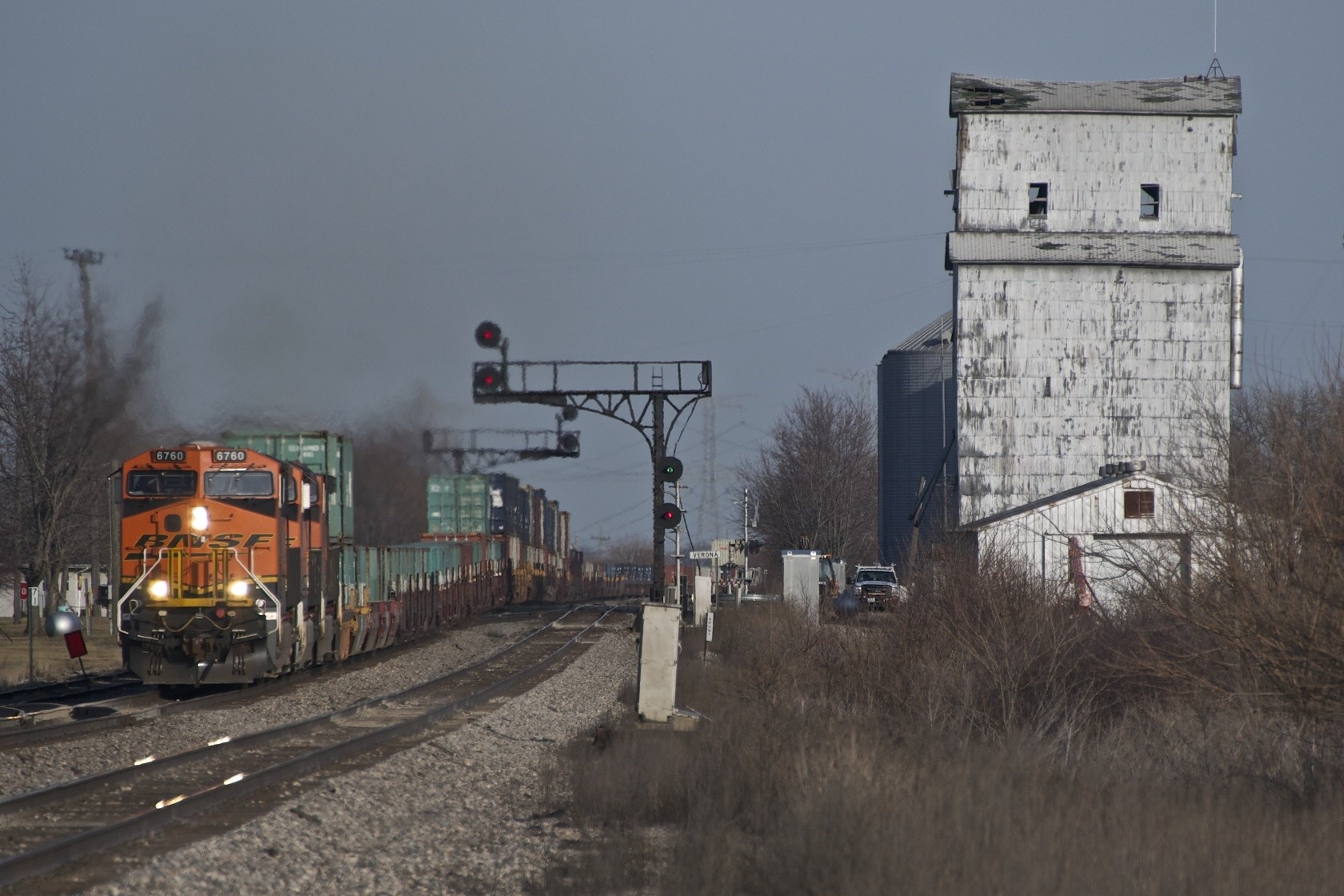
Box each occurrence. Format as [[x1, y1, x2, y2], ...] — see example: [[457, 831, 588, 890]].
[[846, 566, 901, 613]]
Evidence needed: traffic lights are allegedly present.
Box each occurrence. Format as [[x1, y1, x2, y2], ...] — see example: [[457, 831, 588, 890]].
[[749, 540, 762, 554], [477, 366, 503, 392], [655, 503, 682, 529], [475, 320, 503, 350], [656, 457, 684, 482]]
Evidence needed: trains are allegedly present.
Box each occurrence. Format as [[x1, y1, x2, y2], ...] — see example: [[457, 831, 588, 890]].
[[112, 428, 762, 688]]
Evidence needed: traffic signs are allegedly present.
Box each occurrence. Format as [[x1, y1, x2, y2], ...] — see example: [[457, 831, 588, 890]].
[[688, 551, 720, 560]]
